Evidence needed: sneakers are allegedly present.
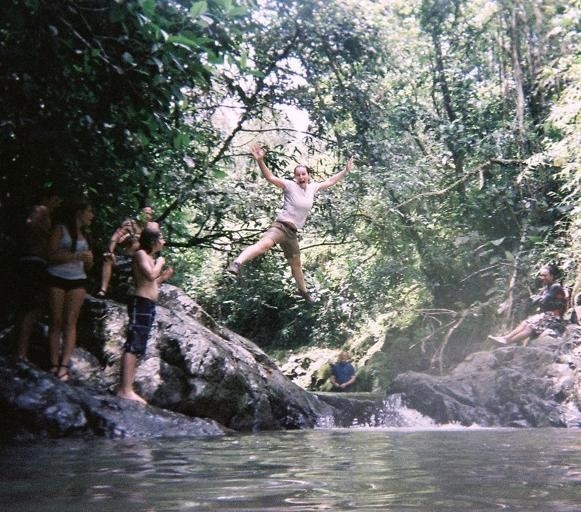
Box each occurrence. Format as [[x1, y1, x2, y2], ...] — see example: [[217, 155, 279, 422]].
[[300, 291, 314, 304], [96, 290, 105, 298], [227, 262, 241, 275]]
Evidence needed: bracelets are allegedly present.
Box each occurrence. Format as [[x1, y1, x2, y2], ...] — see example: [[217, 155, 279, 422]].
[[76, 252, 80, 261]]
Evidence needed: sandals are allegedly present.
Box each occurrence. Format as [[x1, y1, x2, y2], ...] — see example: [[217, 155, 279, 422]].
[[49, 364, 69, 383]]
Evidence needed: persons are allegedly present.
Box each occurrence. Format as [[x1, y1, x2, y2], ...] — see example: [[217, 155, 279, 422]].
[[116, 228, 173, 406], [44, 200, 96, 383], [488, 264, 566, 347], [96, 207, 159, 298], [228, 144, 355, 305], [330, 351, 356, 392], [14, 190, 63, 371]]
